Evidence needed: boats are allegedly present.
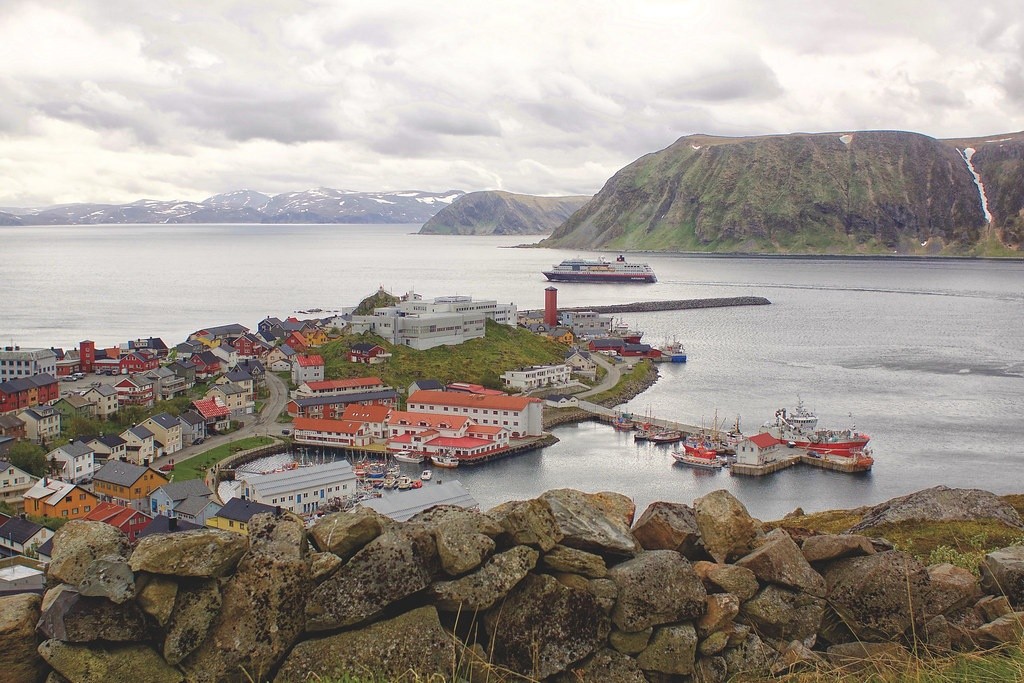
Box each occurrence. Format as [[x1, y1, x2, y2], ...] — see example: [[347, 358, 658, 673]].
[[272, 444, 433, 491], [432, 453, 458, 469], [758, 405, 871, 457], [542, 257, 657, 284], [659, 334, 685, 353], [611, 317, 643, 343], [611, 411, 737, 471]]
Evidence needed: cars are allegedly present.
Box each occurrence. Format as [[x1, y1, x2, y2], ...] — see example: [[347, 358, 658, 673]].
[[95, 367, 128, 376], [193, 438, 204, 445], [58, 374, 73, 382], [73, 373, 85, 379], [158, 464, 174, 471]]
